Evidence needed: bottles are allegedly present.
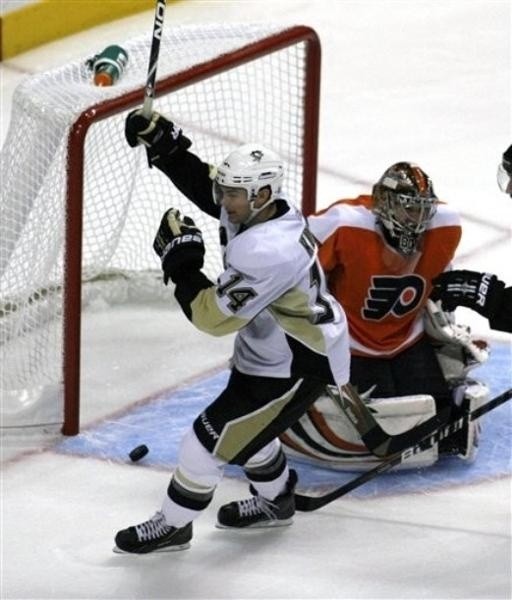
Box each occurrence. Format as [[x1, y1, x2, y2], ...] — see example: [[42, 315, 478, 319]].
[[93, 45, 129, 89]]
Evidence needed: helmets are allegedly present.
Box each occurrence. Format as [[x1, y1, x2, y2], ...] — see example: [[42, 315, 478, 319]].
[[373, 161, 446, 237], [212, 142, 286, 205], [495, 145, 512, 196]]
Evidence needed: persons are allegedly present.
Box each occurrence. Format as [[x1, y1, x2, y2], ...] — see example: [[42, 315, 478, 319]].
[[279, 160, 491, 473], [428, 145, 512, 335], [115, 108, 351, 554]]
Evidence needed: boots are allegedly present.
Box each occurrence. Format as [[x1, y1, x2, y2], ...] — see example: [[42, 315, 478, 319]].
[[216, 469, 298, 526], [113, 510, 194, 554]]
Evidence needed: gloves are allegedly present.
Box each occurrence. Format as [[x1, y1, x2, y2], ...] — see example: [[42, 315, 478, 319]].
[[430, 270, 506, 318], [125, 107, 193, 169], [152, 207, 206, 286]]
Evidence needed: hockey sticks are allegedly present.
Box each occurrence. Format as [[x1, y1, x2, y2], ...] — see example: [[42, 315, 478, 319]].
[[250, 389, 510, 512], [325, 383, 470, 459]]
